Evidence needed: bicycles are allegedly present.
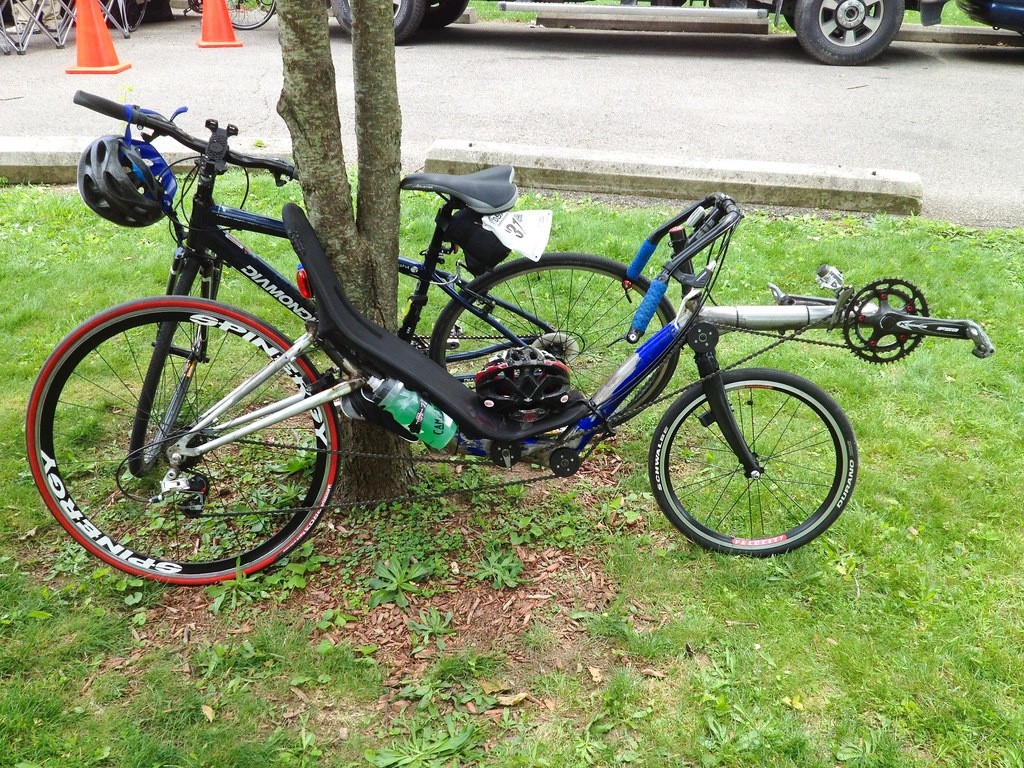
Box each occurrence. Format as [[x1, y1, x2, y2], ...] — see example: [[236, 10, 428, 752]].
[[23, 194, 996, 589], [75, 85, 681, 477], [122, 0, 276, 32]]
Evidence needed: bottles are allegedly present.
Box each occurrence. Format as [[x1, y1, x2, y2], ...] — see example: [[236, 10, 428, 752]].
[[367, 377, 457, 451]]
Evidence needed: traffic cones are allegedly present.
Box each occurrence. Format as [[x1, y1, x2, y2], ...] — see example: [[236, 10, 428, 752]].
[[64, 0, 132, 75], [197, 0, 243, 48]]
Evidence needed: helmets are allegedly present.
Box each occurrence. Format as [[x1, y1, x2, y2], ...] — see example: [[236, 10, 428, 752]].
[[475, 344, 573, 424], [76, 135, 178, 228]]
